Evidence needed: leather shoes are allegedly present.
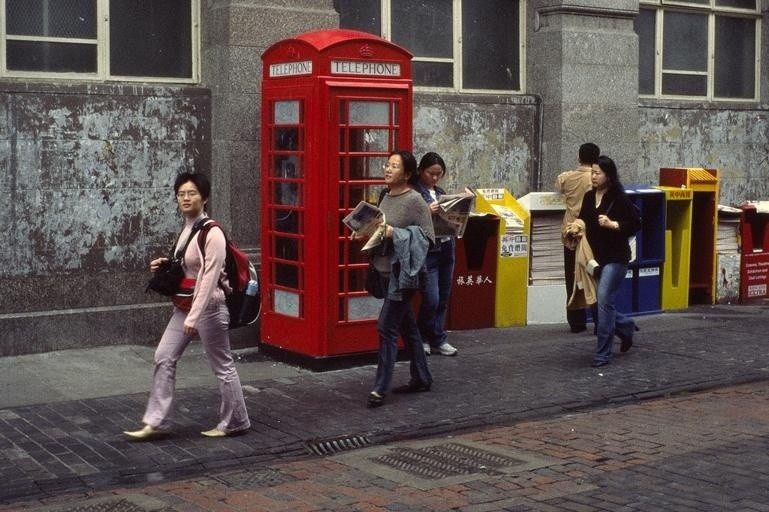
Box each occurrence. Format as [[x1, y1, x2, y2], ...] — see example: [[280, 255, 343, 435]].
[[199, 426, 251, 438], [122, 422, 171, 440]]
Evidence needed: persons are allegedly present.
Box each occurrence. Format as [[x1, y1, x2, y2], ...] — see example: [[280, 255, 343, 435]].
[[123, 172, 252, 440], [554, 142, 600, 336], [350, 150, 435, 408], [417, 152, 459, 356], [571, 155, 639, 368]]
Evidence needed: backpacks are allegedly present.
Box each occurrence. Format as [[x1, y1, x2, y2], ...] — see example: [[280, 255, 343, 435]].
[[197, 221, 262, 329]]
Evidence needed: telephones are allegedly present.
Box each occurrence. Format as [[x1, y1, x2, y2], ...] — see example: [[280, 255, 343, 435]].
[[281, 159, 303, 205]]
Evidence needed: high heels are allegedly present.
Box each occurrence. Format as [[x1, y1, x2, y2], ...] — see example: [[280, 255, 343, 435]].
[[366, 390, 386, 407], [392, 377, 434, 394], [591, 359, 608, 367], [620, 320, 640, 352]]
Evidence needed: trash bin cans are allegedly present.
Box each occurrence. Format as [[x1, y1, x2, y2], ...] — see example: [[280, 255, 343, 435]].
[[446, 216, 500, 328], [741, 206, 769, 301]]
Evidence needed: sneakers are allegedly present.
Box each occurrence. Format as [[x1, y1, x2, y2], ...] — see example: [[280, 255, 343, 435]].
[[421, 342, 457, 356]]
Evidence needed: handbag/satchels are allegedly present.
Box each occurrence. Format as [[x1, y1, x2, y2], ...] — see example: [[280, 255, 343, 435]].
[[364, 266, 391, 300], [149, 258, 198, 316]]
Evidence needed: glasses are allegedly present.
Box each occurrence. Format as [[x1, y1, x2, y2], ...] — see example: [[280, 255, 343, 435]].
[[382, 162, 401, 169]]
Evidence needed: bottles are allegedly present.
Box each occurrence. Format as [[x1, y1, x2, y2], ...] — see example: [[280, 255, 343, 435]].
[[245, 279, 259, 296]]
